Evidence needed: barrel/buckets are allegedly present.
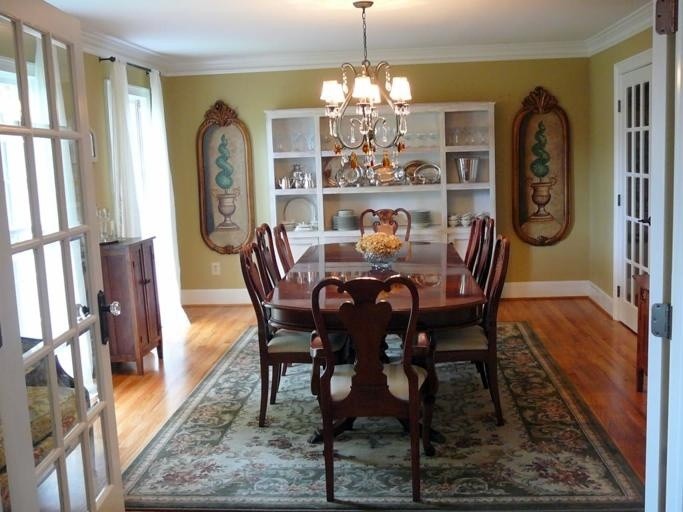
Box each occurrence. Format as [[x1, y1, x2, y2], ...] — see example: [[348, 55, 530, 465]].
[[456, 157, 480, 183]]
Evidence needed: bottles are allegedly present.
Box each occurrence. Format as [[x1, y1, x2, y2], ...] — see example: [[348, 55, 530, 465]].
[[96, 208, 117, 242], [292, 164, 314, 188]]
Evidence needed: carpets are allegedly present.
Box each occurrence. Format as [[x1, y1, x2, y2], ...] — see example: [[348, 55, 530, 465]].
[[120, 321, 644, 512]]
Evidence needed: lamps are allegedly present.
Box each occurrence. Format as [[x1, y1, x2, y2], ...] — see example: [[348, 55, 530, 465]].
[[320, 1, 413, 169]]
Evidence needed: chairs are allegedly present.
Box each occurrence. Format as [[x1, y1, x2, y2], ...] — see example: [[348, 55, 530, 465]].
[[254, 223, 296, 391], [406, 235, 509, 427], [309, 274, 440, 502], [359, 209, 412, 241], [464, 213, 495, 389], [242, 243, 352, 428]]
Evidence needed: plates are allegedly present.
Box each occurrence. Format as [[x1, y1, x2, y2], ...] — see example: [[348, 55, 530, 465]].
[[365, 162, 404, 185], [402, 160, 430, 179], [331, 208, 433, 231], [321, 155, 363, 186], [282, 197, 316, 225], [414, 165, 440, 184]]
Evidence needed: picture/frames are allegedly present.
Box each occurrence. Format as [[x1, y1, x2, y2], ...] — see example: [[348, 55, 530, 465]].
[[196, 100, 255, 254], [512, 86, 569, 246]]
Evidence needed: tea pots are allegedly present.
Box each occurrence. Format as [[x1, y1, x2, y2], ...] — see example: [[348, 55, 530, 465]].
[[278, 176, 294, 189]]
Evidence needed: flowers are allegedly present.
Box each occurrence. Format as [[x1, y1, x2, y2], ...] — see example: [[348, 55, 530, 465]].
[[356, 232, 405, 268]]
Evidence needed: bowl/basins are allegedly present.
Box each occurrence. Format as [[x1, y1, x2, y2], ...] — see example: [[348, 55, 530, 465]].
[[448, 213, 490, 228], [281, 222, 296, 231]]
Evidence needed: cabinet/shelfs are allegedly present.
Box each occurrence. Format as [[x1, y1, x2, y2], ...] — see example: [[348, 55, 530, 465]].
[[263, 102, 496, 288], [100, 236, 163, 375], [636, 274, 650, 393]]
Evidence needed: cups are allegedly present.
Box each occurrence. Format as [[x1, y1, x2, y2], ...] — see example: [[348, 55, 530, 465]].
[[405, 130, 489, 144]]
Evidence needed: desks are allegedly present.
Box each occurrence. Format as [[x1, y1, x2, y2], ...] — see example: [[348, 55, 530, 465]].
[[262, 241, 487, 441]]
